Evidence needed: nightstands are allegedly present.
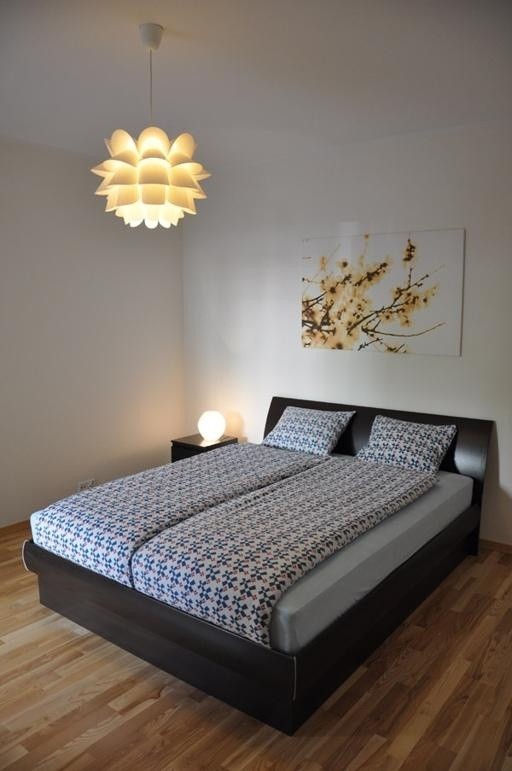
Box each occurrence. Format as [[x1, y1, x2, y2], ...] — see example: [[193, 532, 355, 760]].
[[171, 433, 238, 463]]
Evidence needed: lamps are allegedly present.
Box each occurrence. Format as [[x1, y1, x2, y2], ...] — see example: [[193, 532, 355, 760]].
[[197, 411, 227, 442], [199, 440, 220, 447], [90, 23, 212, 230]]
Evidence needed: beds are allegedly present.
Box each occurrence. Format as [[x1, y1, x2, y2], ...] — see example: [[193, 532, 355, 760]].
[[21, 395, 494, 736]]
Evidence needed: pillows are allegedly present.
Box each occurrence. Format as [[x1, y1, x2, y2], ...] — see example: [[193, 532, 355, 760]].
[[261, 405, 357, 456], [355, 415, 457, 483]]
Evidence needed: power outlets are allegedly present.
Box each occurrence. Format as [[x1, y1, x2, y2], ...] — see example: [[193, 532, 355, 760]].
[[78, 480, 91, 491]]
